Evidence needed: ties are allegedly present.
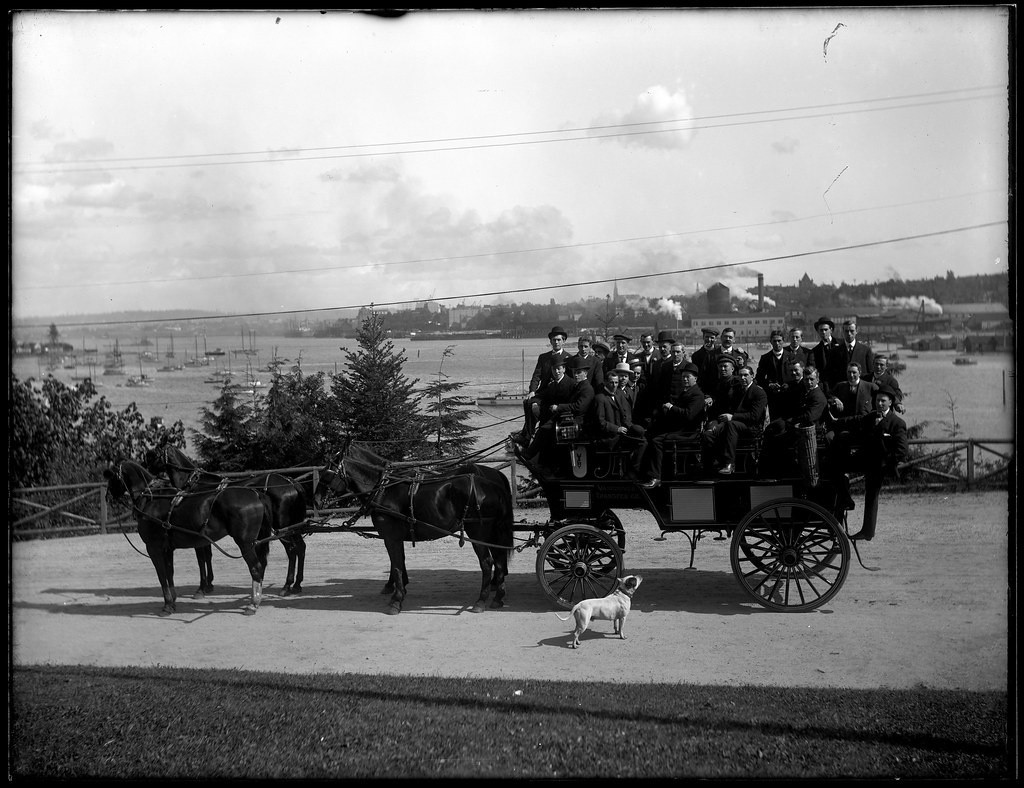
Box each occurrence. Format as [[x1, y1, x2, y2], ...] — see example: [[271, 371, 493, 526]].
[[741, 386, 746, 394], [849, 345, 853, 358], [619, 356, 625, 362], [792, 350, 795, 355]]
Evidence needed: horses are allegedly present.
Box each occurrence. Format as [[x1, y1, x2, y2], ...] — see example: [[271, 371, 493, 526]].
[[314, 435, 515, 616], [104, 433, 305, 616]]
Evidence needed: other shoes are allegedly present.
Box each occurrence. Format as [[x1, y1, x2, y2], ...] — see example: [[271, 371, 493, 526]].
[[513, 443, 527, 465], [851, 531, 873, 541], [509, 434, 529, 446]]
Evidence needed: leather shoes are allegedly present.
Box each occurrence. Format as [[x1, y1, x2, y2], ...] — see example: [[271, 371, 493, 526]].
[[718, 463, 736, 474], [640, 478, 662, 488]]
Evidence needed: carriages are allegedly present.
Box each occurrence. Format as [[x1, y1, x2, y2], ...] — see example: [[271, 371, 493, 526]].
[[103, 432, 853, 616]]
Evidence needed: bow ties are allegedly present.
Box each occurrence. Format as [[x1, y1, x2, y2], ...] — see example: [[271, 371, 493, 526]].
[[645, 353, 651, 355], [776, 353, 781, 356], [874, 375, 880, 381]]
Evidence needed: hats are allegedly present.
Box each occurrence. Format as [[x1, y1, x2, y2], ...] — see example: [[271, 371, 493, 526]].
[[628, 359, 644, 367], [877, 383, 897, 399], [567, 356, 591, 370], [701, 327, 721, 335], [679, 363, 699, 374], [612, 362, 634, 374], [769, 418, 786, 436], [827, 395, 844, 421], [613, 334, 633, 340], [717, 354, 737, 366], [623, 424, 647, 441], [814, 316, 835, 330], [548, 325, 568, 340], [548, 354, 567, 366], [655, 331, 675, 343], [591, 340, 610, 352]]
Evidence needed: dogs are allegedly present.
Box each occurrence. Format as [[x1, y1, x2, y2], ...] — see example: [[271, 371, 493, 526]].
[[555, 575, 642, 649]]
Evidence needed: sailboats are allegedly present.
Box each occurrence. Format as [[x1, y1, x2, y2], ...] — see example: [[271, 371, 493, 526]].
[[60, 326, 278, 389]]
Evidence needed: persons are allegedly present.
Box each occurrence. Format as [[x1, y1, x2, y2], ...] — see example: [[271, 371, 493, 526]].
[[511, 315, 909, 542]]
